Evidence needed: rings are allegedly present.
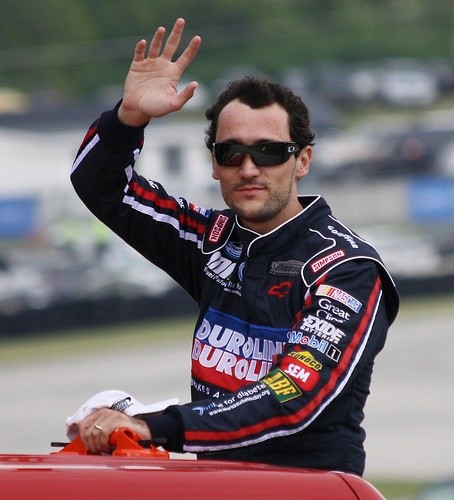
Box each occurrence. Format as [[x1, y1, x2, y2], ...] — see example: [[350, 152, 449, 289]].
[[94, 425, 102, 430]]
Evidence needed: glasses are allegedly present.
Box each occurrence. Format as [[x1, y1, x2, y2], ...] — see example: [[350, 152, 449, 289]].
[[211, 141, 300, 168]]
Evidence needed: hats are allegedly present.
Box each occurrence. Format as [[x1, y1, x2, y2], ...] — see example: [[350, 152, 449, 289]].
[[65, 389, 181, 442]]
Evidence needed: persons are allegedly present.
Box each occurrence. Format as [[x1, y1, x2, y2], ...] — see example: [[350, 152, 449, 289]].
[[70, 18, 399, 480]]
